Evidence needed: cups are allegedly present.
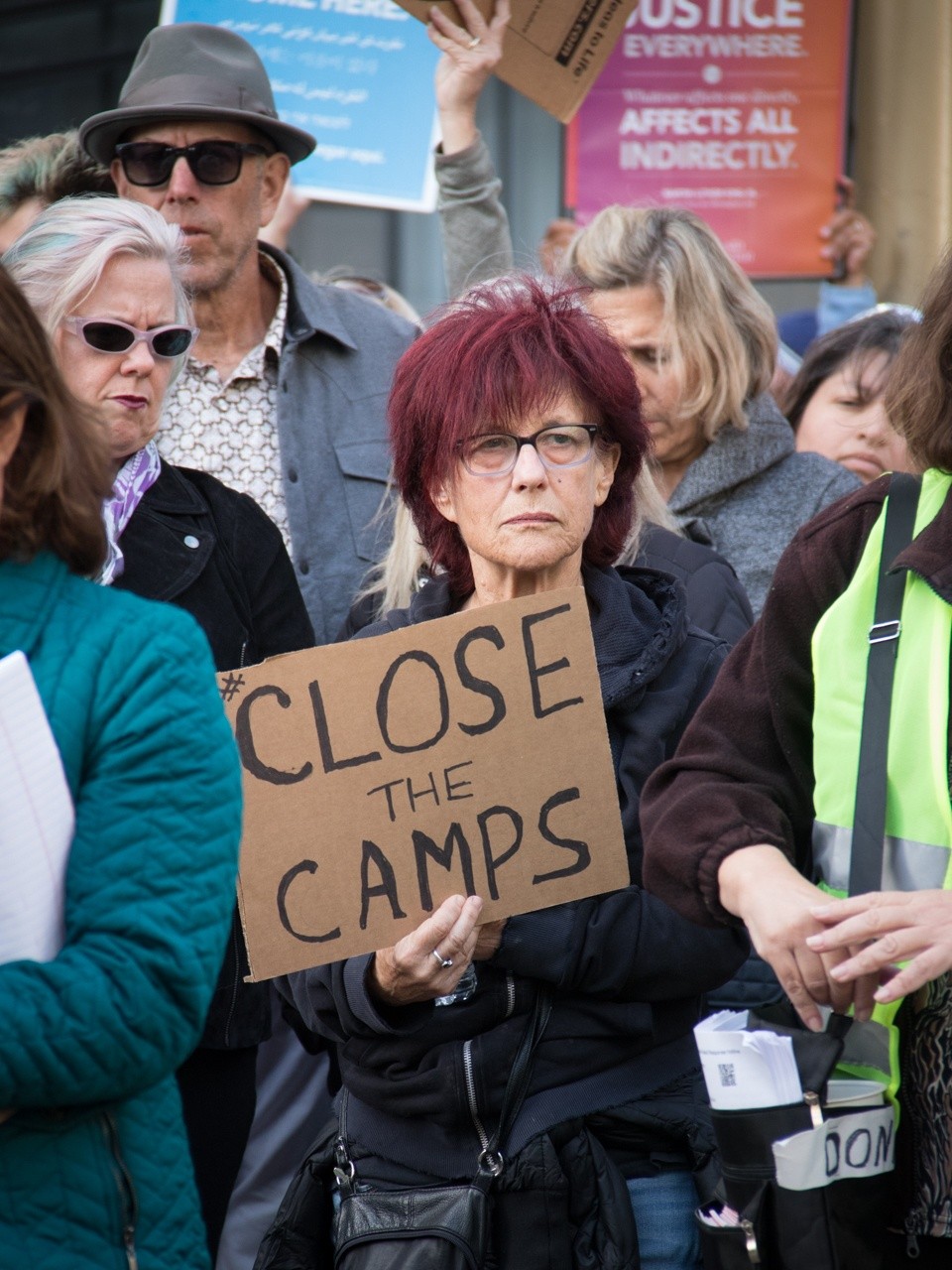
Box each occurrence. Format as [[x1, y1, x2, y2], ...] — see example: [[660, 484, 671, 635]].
[[822, 1078, 888, 1109]]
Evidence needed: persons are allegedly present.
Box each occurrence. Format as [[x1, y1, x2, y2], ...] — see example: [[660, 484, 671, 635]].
[[0, 0, 951, 647], [271, 278, 752, 1270], [637, 253, 952, 1270], [0, 196, 319, 1270], [0, 259, 244, 1269]]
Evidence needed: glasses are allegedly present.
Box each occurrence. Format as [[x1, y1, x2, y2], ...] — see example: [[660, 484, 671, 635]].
[[446, 423, 601, 477], [116, 140, 281, 187], [62, 315, 202, 359]]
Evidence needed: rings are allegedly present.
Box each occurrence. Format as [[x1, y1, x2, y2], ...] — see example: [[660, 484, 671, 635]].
[[466, 37, 481, 49], [433, 951, 453, 969], [853, 221, 864, 229]]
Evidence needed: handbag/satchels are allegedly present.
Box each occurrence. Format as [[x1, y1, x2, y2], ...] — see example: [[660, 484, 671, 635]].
[[708, 1008, 895, 1269], [334, 1187, 488, 1270]]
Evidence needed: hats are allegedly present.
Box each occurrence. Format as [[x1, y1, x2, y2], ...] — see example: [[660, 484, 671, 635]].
[[78, 23, 317, 167]]
